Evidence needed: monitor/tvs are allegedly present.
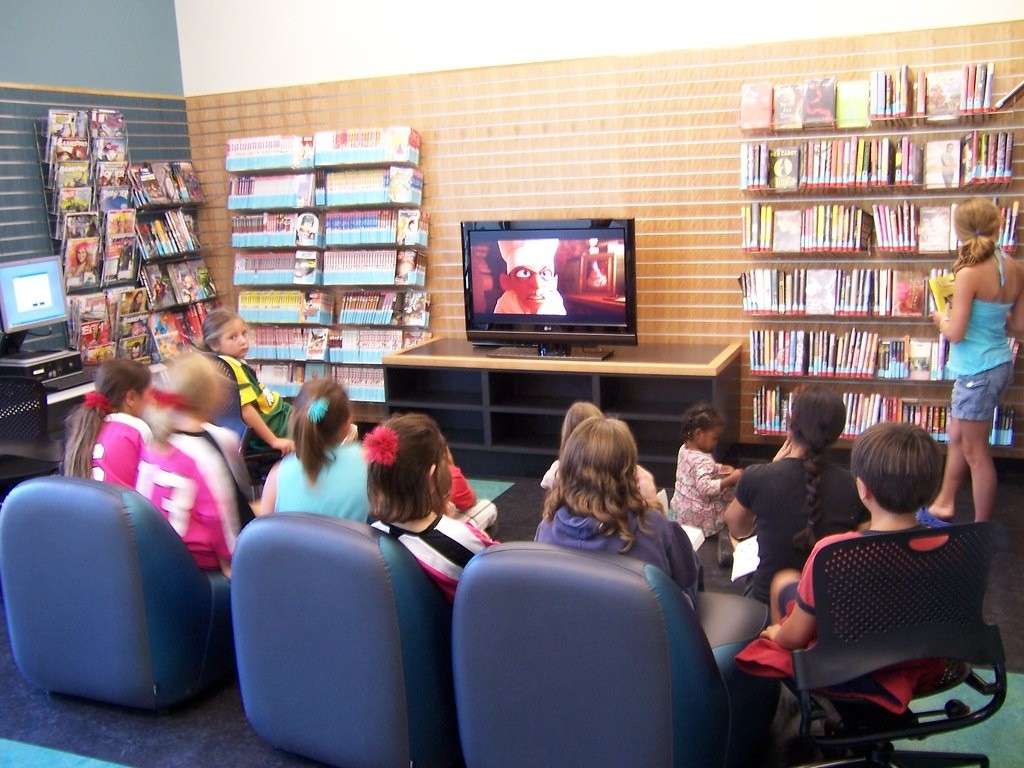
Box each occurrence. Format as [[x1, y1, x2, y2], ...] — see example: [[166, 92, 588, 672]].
[[0, 255, 71, 364], [461, 217, 638, 361]]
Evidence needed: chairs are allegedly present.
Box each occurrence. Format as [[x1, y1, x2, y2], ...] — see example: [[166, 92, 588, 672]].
[[0, 351, 1011, 767]]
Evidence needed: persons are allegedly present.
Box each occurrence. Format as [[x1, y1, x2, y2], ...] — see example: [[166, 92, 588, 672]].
[[243, 381, 379, 532], [57, 359, 239, 581], [144, 353, 253, 562], [760, 421, 960, 745], [533, 416, 706, 615], [670, 403, 745, 538], [724, 385, 872, 603], [538, 401, 659, 512], [196, 309, 301, 456], [362, 413, 504, 668], [912, 195, 1024, 532], [436, 445, 498, 538]]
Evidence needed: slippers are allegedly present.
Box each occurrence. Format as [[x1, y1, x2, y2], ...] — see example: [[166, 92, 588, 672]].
[[917, 505, 956, 527]]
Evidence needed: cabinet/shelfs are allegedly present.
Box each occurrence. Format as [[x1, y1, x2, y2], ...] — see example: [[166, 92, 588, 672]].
[[34, 109, 150, 364], [741, 113, 1024, 447], [130, 160, 223, 357], [380, 338, 744, 486], [228, 147, 429, 403]]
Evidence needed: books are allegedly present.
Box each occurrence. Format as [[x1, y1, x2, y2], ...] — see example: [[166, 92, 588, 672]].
[[215, 123, 434, 406], [42, 107, 228, 372], [738, 63, 1024, 446]]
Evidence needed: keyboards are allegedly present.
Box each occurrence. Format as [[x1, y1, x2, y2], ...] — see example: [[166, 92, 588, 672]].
[[44, 371, 95, 395]]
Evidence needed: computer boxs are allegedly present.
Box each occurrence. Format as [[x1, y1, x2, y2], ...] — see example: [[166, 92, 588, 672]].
[[0, 348, 83, 386]]
